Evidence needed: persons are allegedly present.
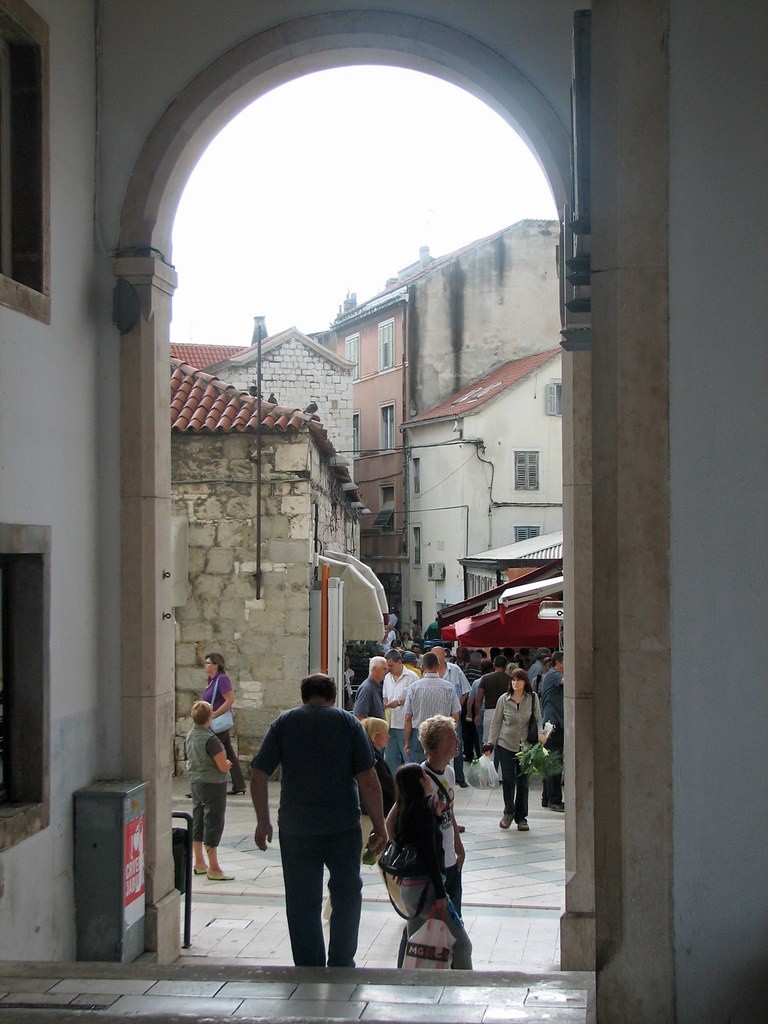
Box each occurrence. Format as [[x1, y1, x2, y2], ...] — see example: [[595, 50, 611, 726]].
[[184, 700, 233, 881], [344, 609, 564, 831], [250, 672, 389, 968], [378, 715, 474, 970], [186, 653, 247, 799]]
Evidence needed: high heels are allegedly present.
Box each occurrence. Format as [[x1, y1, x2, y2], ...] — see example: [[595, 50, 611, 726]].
[[227, 789, 246, 795], [186, 793, 192, 798]]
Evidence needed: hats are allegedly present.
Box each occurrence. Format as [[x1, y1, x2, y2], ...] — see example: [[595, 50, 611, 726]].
[[402, 653, 419, 662]]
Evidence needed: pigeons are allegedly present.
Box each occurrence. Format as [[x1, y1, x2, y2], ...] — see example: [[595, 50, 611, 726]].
[[249, 386, 318, 414]]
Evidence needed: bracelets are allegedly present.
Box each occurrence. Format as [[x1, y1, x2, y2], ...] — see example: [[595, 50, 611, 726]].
[[398, 699, 400, 706]]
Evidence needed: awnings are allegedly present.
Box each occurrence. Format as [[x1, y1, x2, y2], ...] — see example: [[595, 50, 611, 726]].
[[318, 550, 390, 641]]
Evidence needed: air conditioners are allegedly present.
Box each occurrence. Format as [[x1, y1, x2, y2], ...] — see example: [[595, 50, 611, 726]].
[[428, 562, 445, 582]]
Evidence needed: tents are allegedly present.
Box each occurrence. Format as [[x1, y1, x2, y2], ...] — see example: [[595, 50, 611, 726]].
[[436, 558, 565, 652]]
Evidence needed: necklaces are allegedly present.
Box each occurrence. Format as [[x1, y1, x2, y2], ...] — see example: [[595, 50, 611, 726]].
[[206, 680, 214, 689]]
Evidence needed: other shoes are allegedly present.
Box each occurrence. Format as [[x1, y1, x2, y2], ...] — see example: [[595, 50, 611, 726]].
[[194, 868, 207, 874], [548, 801, 564, 811], [542, 802, 547, 806], [207, 872, 234, 880], [500, 813, 514, 828], [456, 780, 468, 788], [518, 821, 529, 831]]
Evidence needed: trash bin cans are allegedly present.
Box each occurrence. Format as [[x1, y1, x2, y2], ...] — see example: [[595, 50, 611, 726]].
[[172, 827, 188, 895]]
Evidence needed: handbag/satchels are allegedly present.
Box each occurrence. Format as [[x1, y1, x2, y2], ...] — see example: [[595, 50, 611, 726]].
[[467, 753, 499, 789], [401, 903, 456, 968], [209, 710, 234, 734], [377, 802, 431, 878], [527, 693, 538, 743]]
